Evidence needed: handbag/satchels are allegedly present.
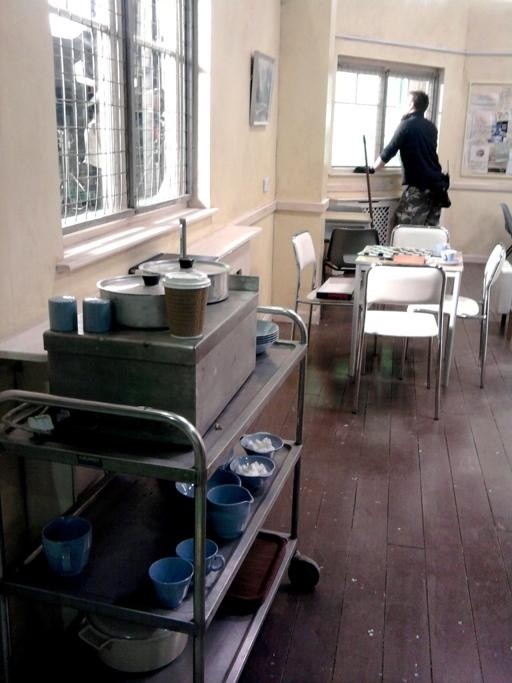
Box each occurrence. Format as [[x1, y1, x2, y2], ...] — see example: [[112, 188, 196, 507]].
[[439, 172, 451, 208]]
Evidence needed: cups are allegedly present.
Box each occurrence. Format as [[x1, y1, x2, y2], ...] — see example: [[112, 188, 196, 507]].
[[174, 537, 227, 576], [161, 270, 211, 339], [431, 243, 447, 256], [440, 249, 456, 261], [48, 294, 77, 332], [149, 557, 193, 606], [80, 297, 110, 332], [206, 483, 255, 541], [42, 513, 90, 579]]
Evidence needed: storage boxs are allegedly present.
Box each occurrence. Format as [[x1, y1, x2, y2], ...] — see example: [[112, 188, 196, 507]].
[[42, 290, 266, 453]]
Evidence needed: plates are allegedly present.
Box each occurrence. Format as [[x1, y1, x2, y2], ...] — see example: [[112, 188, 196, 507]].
[[256, 318, 279, 354], [437, 258, 460, 265]]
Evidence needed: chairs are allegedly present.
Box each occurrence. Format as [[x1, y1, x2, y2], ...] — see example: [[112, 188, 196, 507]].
[[499, 202, 512, 260], [322, 229, 380, 285], [389, 225, 450, 248], [346, 263, 446, 418], [396, 241, 506, 389], [291, 230, 365, 377]]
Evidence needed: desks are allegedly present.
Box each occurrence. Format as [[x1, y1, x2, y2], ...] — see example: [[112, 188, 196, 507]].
[[342, 248, 464, 389]]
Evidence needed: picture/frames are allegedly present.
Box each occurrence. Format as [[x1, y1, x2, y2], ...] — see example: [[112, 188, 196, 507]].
[[249, 50, 276, 128]]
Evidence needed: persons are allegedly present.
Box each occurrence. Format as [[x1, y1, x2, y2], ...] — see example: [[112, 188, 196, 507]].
[[373, 91, 445, 226]]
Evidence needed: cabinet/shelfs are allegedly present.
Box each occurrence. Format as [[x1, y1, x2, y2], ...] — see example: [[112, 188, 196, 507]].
[[0, 302, 322, 681]]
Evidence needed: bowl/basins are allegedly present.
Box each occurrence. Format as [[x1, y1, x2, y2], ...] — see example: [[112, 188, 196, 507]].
[[99, 274, 168, 329], [228, 455, 274, 488], [137, 257, 230, 303], [240, 430, 285, 462], [175, 470, 243, 510]]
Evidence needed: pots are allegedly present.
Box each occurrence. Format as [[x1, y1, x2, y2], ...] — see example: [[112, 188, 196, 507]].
[[77, 613, 188, 673]]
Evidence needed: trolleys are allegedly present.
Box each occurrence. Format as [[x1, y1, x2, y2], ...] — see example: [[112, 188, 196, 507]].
[[0, 305, 319, 683]]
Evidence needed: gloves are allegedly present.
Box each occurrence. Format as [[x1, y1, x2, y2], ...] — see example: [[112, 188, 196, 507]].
[[354, 165, 375, 174]]
